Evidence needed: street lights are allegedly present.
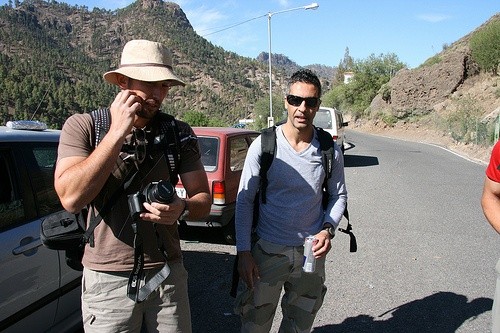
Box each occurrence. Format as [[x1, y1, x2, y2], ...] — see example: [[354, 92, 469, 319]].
[[267, 3, 319, 128]]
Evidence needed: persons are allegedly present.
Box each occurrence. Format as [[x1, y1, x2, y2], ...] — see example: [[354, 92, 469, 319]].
[[234, 68, 348, 333], [480, 138, 500, 333], [54, 39, 213, 333]]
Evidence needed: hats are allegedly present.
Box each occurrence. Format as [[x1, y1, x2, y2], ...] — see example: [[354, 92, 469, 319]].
[[103, 39, 187, 87]]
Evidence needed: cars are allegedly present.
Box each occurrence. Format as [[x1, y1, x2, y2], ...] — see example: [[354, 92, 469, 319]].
[[0, 125, 84, 333], [175, 127, 262, 228]]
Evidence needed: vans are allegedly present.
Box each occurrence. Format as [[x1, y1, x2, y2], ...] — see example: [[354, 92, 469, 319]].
[[312, 107, 349, 155]]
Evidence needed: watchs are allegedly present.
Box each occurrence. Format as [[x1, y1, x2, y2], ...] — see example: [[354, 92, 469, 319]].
[[322, 226, 336, 239], [175, 198, 191, 225]]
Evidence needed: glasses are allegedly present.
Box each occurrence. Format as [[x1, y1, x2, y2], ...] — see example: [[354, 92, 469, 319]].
[[286, 94, 322, 108]]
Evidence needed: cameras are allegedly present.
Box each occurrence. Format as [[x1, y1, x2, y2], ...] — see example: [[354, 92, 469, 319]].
[[125, 181, 174, 220]]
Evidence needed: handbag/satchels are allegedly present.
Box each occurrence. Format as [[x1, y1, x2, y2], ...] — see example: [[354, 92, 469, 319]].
[[65, 214, 103, 272], [40, 208, 91, 250]]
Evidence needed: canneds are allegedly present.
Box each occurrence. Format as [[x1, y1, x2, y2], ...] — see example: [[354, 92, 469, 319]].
[[302, 237, 317, 272]]
